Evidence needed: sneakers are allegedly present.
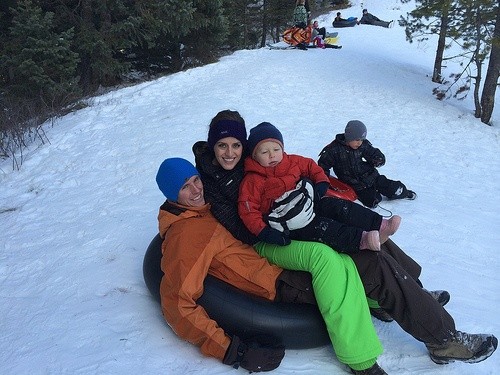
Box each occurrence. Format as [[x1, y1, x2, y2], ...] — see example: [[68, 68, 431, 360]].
[[426, 329, 498, 365], [360, 229, 381, 252], [378, 214, 400, 245], [422, 287, 449, 306]]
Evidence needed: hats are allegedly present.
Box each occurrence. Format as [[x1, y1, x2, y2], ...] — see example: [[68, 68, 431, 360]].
[[247, 122, 284, 159], [344, 120, 366, 141], [156, 158, 202, 203], [208, 110, 247, 152]]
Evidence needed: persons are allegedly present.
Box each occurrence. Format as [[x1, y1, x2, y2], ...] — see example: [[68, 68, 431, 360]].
[[293, 0, 307, 28], [317, 120, 417, 209], [238, 122, 402, 255], [191, 109, 451, 375], [357, 9, 395, 29], [334, 12, 359, 26], [310, 21, 342, 49], [155, 158, 499, 372]]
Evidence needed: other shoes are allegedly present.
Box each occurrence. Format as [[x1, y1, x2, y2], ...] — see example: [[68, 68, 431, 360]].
[[406, 190, 418, 201], [370, 307, 394, 322], [351, 363, 388, 375]]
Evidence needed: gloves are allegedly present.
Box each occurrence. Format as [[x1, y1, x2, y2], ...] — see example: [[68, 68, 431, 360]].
[[371, 158, 384, 167], [261, 228, 291, 246], [314, 182, 329, 201], [223, 335, 287, 372], [268, 177, 316, 232]]
